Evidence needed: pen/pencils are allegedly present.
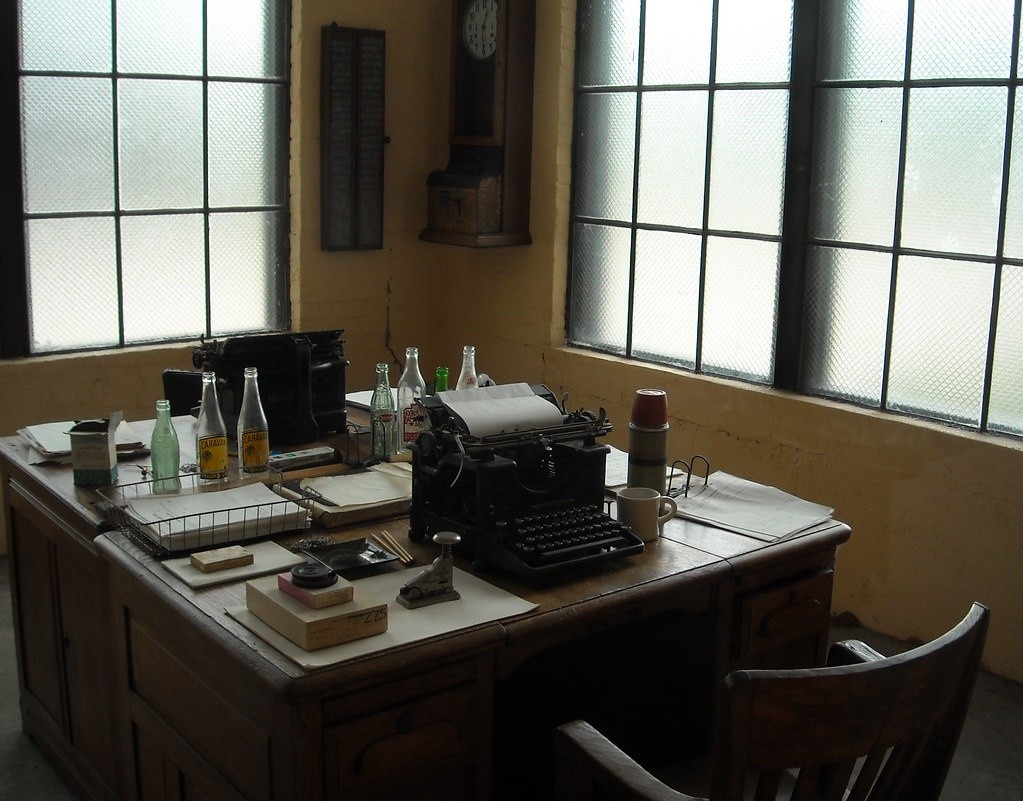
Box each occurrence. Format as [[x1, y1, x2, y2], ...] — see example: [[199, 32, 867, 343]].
[[369, 530, 413, 565]]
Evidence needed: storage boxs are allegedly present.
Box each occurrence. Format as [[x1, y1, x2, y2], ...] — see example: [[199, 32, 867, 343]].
[[63, 410, 124, 487]]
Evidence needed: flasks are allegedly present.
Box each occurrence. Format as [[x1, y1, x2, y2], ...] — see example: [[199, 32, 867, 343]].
[[627, 389, 670, 516]]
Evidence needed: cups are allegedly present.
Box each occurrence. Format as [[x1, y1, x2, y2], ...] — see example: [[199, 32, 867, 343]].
[[617, 487, 678, 542]]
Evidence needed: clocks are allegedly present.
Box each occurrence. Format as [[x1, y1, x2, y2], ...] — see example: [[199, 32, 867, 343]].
[[464, 0, 499, 59]]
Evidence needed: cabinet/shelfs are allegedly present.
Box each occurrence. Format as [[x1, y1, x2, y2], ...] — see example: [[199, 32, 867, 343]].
[[0, 373, 851, 801]]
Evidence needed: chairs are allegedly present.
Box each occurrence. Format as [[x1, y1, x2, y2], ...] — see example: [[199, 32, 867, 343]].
[[548, 600, 991, 801]]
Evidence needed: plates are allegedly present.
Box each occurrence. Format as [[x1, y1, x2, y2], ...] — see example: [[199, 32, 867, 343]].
[[300, 537, 399, 573]]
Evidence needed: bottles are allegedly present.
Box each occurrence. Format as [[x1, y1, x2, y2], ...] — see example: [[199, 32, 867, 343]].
[[371, 363, 396, 459], [237, 367, 269, 480], [455, 345, 478, 390], [150, 399, 182, 495], [396, 347, 426, 454], [435, 366, 449, 392], [195, 371, 229, 486]]
